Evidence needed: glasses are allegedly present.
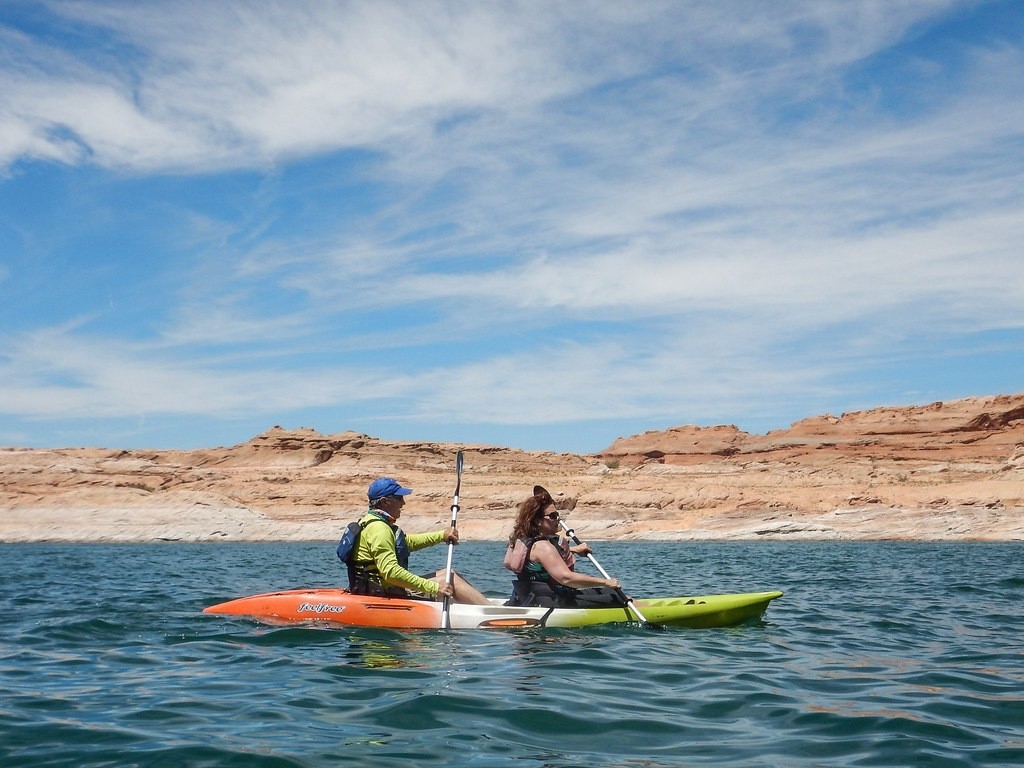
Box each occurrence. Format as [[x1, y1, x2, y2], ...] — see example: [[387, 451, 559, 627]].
[[385, 495, 403, 501], [543, 511, 559, 519]]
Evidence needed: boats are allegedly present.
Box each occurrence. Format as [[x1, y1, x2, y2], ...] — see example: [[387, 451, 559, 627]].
[[200, 587, 784, 630]]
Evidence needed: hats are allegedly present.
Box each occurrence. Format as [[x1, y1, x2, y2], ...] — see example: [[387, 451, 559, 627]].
[[367, 477, 413, 500]]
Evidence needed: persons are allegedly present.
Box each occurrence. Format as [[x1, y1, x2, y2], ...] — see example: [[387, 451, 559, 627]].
[[504, 494, 706, 608], [344, 478, 494, 606]]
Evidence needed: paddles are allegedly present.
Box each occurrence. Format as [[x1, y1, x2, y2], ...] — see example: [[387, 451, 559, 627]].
[[441, 451, 465, 630], [532, 484, 667, 631]]
